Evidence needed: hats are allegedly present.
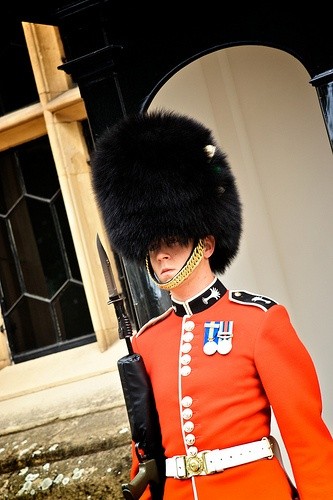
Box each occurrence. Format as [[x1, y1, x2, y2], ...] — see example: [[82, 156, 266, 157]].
[[91, 116, 242, 274]]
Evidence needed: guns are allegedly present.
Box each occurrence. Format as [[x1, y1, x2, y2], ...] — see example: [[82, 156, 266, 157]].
[[96, 234, 166, 500]]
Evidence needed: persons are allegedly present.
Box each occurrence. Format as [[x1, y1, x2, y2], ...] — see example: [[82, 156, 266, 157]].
[[89, 112, 333, 500]]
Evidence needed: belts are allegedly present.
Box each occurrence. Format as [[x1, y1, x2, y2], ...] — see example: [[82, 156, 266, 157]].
[[165, 437, 272, 482]]
